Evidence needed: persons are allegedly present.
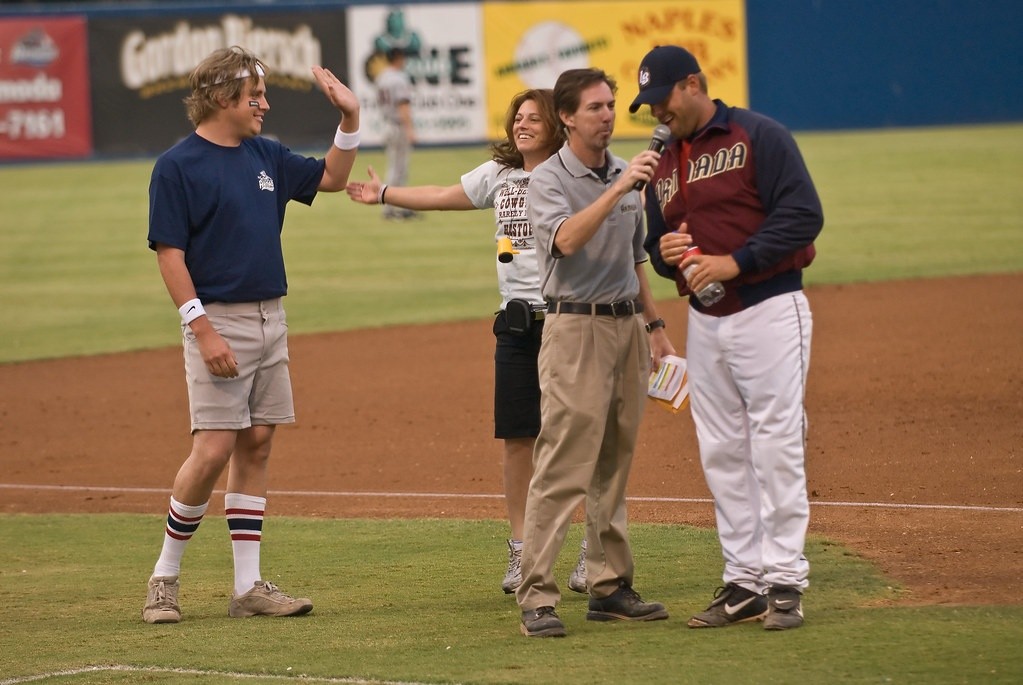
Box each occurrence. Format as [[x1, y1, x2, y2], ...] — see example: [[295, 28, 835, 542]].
[[376, 48, 419, 217], [629, 45, 824, 630], [143, 45, 361, 623], [346, 87, 589, 592], [516, 67, 677, 636]]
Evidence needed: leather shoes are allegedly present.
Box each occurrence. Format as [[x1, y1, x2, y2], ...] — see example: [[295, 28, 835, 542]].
[[520, 607, 567, 637], [586, 580, 668, 621]]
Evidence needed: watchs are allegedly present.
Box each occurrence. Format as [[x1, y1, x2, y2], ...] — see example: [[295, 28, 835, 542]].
[[645, 317, 665, 333]]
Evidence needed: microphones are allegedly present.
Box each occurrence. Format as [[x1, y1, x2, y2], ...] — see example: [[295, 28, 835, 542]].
[[632, 123, 672, 191]]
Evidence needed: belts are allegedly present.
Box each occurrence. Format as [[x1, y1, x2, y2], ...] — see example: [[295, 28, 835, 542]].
[[531, 308, 547, 321], [547, 299, 643, 319]]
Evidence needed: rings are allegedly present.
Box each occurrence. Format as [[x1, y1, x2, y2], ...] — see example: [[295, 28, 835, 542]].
[[361, 187, 363, 191]]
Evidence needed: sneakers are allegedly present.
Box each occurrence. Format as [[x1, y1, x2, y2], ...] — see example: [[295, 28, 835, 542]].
[[501, 539, 523, 593], [229, 581, 313, 618], [686, 583, 771, 628], [143, 573, 181, 624], [762, 582, 804, 630], [568, 546, 586, 592]]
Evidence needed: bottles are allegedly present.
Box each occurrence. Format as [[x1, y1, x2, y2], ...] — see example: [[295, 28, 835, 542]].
[[671, 230, 726, 307]]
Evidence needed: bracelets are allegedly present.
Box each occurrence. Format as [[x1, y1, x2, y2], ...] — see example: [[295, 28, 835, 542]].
[[334, 125, 361, 149], [178, 298, 206, 324], [377, 184, 388, 204]]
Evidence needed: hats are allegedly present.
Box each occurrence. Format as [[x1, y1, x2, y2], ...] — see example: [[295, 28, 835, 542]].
[[629, 45, 701, 113]]
